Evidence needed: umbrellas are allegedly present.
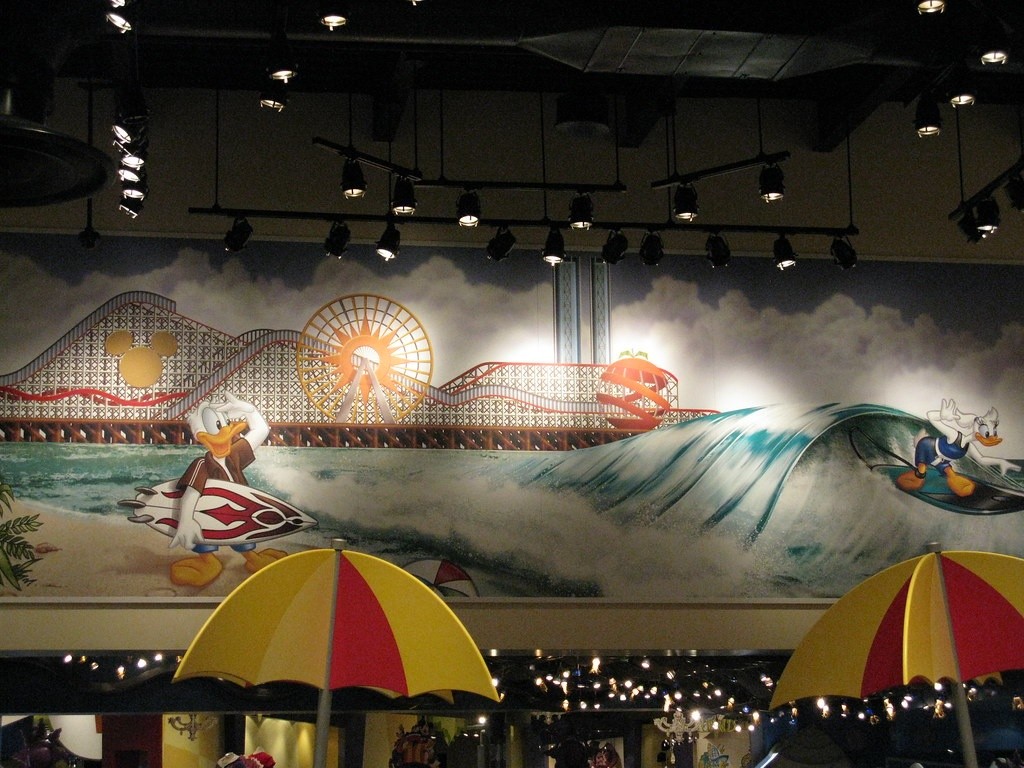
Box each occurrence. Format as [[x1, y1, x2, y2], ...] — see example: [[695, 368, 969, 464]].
[[171, 549, 501, 707], [768, 551, 1023, 714]]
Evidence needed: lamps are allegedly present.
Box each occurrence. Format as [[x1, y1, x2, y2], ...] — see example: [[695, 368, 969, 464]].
[[72, 0, 1023, 274]]
